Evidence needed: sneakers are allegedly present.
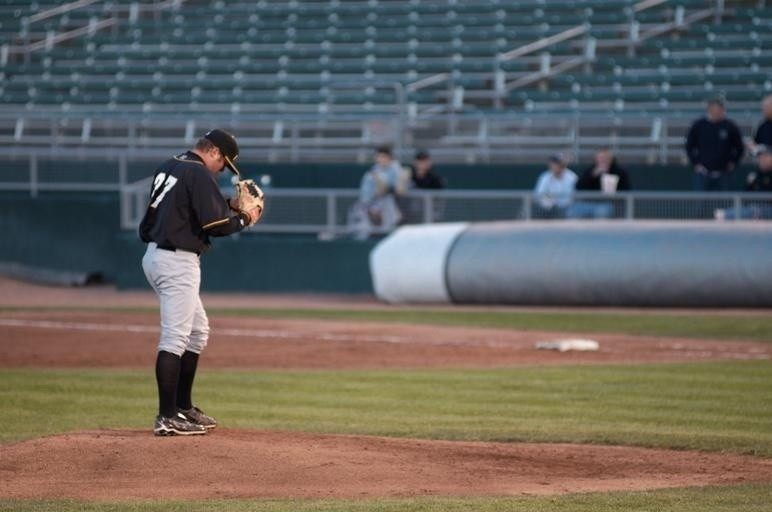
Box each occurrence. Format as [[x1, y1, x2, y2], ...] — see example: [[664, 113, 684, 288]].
[[153, 406, 217, 436]]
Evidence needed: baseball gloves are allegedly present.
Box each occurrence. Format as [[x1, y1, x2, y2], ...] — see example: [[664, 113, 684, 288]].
[[226, 179, 264, 226]]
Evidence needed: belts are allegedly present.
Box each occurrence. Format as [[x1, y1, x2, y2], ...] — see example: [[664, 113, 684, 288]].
[[156, 243, 176, 252]]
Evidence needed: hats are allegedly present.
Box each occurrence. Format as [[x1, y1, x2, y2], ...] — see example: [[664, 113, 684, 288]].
[[204, 129, 240, 175]]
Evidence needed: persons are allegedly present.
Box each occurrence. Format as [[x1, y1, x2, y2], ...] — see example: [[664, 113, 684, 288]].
[[533, 146, 632, 218], [355, 148, 444, 242], [686, 95, 743, 219], [139, 129, 265, 435], [743, 91, 772, 218]]
